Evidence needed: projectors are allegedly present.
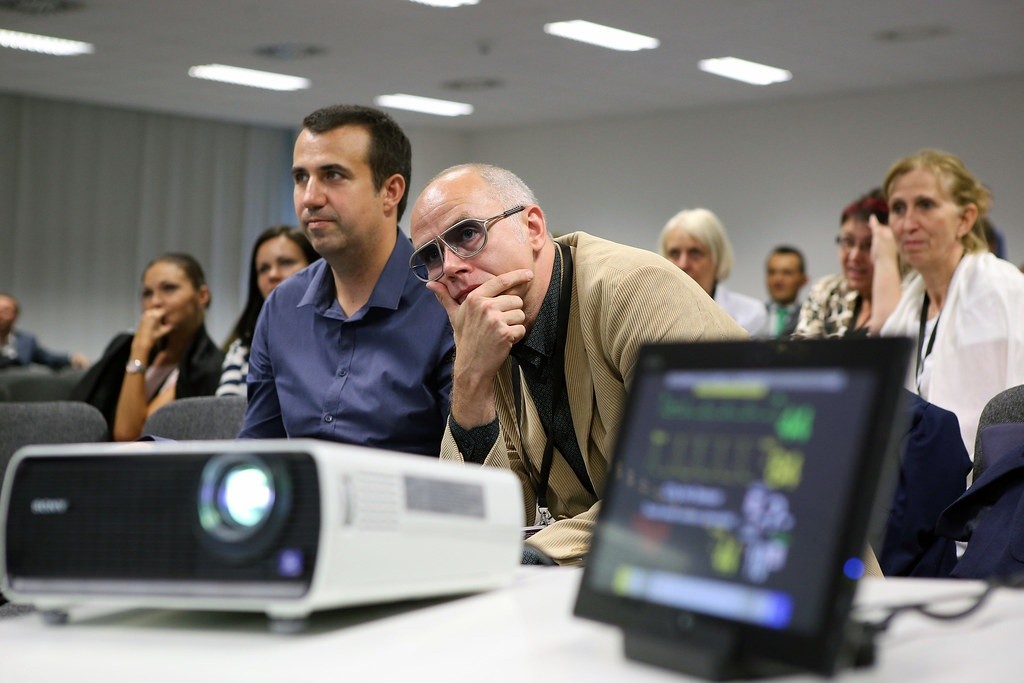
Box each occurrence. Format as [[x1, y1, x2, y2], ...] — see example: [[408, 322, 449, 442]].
[[0, 435, 524, 630]]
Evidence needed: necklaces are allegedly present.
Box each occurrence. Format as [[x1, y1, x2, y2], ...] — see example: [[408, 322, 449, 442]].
[[846, 314, 871, 336], [915, 246, 967, 399]]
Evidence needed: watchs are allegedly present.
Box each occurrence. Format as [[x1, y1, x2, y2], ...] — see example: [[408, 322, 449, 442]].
[[125, 358, 146, 372]]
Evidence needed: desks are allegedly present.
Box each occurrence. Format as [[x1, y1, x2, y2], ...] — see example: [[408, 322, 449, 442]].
[[0, 566, 1024, 683]]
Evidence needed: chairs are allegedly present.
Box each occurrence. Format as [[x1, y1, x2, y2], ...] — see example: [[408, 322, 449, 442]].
[[874, 385, 1024, 581], [0, 366, 250, 500]]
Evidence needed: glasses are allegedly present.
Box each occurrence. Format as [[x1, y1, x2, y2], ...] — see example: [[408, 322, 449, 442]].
[[837, 235, 870, 252], [409, 205, 525, 282]]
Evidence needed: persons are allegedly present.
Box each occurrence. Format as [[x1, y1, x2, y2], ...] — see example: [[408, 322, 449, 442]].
[[0, 105, 1024, 567]]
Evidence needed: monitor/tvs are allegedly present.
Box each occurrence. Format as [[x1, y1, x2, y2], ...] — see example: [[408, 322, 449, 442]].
[[576, 333, 917, 680]]
